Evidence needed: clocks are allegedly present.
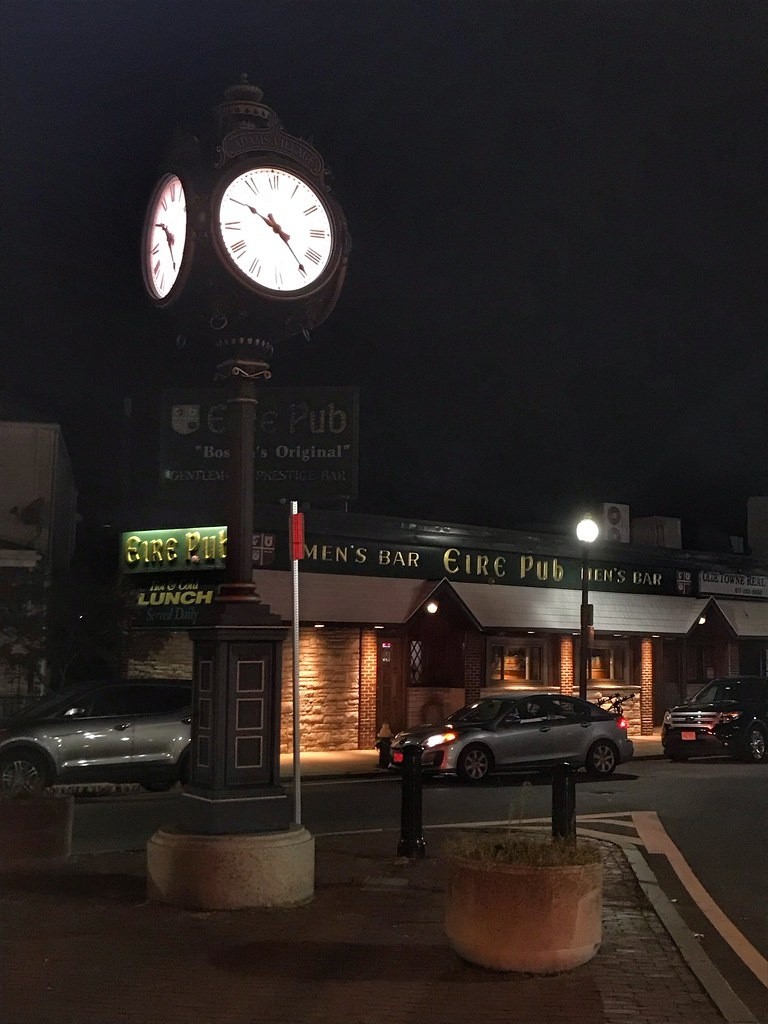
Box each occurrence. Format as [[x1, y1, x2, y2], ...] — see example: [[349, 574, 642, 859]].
[[137, 169, 199, 309], [207, 155, 345, 305]]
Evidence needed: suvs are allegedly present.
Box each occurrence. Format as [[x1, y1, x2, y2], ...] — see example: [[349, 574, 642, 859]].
[[0, 674, 200, 792], [664, 672, 768, 765]]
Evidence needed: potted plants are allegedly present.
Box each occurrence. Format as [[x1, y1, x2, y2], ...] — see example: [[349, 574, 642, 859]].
[[440, 781, 604, 974]]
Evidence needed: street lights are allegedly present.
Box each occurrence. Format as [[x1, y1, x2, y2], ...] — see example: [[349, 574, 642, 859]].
[[574, 509, 600, 699]]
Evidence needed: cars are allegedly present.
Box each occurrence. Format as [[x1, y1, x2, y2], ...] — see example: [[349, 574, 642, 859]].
[[384, 694, 633, 783]]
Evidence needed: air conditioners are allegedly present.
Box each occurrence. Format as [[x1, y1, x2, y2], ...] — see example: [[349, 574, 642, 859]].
[[600, 503, 630, 544]]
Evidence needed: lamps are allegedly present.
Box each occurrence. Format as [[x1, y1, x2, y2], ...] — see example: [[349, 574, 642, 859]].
[[426, 596, 440, 614]]
